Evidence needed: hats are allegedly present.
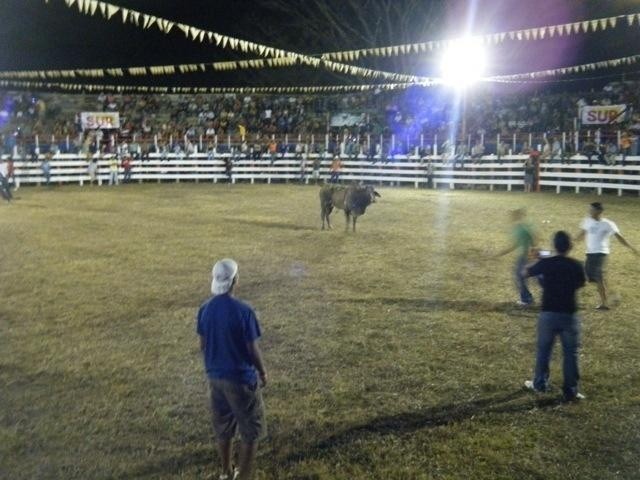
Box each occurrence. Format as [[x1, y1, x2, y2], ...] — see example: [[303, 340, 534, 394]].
[[209, 258, 239, 296]]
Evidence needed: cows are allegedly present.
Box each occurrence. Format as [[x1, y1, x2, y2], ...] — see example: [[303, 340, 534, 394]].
[[319, 182, 381, 233]]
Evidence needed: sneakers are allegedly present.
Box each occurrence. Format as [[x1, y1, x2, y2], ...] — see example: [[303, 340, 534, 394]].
[[524, 379, 586, 401]]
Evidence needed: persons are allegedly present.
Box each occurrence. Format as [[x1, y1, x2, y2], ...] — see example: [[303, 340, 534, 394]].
[[488, 207, 539, 307], [570, 202, 639, 311], [516, 228, 587, 400], [196, 256, 268, 479]]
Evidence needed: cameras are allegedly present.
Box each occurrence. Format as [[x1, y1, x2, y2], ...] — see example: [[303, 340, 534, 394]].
[[538, 249, 553, 258]]
